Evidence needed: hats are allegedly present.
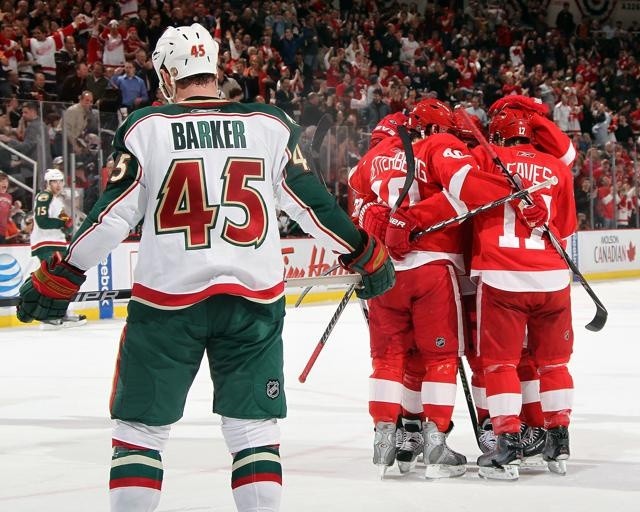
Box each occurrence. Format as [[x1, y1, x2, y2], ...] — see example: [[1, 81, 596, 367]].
[[562, 75, 578, 96]]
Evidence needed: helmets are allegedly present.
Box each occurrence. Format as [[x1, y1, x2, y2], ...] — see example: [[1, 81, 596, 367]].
[[488, 107, 534, 145], [150, 21, 220, 85], [371, 97, 483, 139], [44, 168, 64, 182]]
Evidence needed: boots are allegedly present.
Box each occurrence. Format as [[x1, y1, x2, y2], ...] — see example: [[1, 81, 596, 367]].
[[372, 414, 570, 468]]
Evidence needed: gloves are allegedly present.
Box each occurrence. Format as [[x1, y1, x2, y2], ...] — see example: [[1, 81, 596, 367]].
[[337, 226, 397, 301], [384, 207, 422, 262], [488, 94, 550, 115], [508, 172, 549, 229], [16, 251, 88, 324]]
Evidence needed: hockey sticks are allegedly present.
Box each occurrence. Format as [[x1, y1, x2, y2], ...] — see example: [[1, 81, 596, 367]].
[[454, 104, 607, 331], [295, 175, 558, 310], [299, 125, 414, 381]]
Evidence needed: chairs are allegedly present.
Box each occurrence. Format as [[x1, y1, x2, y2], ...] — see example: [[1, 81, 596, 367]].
[[0, 71, 153, 199]]
